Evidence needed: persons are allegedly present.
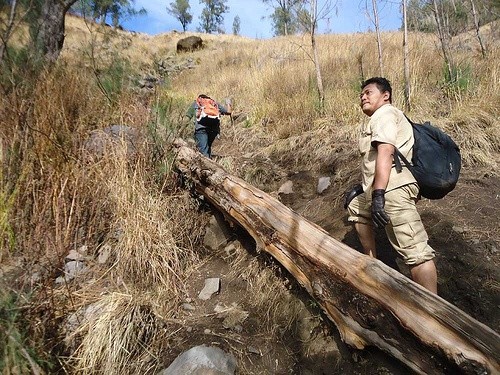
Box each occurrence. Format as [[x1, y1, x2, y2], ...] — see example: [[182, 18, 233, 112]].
[[346, 75, 439, 299], [182, 93, 232, 158]]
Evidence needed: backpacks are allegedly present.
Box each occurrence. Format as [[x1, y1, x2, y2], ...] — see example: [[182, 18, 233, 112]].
[[196, 96, 220, 122], [395, 112, 461, 199]]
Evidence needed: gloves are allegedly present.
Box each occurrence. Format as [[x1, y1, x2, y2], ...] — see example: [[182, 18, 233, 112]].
[[344, 183, 364, 209], [370, 189, 390, 229]]
